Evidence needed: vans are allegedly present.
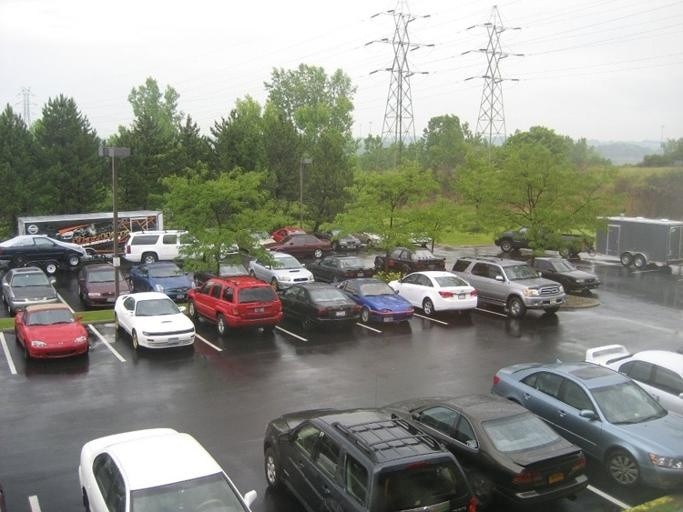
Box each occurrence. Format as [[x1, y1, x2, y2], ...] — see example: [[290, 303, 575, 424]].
[[122, 230, 226, 265]]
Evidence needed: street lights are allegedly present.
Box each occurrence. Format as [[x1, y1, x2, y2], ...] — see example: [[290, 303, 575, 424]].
[[298, 151, 312, 226], [98, 145, 131, 298]]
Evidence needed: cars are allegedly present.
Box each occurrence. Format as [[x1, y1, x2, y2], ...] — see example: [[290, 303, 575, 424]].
[[187, 273, 284, 336], [276, 281, 363, 333], [491, 358, 683, 487], [0, 267, 58, 317], [113, 292, 196, 353], [387, 270, 478, 318], [235, 224, 434, 260], [129, 261, 195, 302], [76, 426, 257, 512], [584, 344, 682, 415], [334, 276, 415, 325], [523, 256, 601, 294], [77, 264, 131, 309], [0, 485, 6, 511], [13, 303, 90, 362], [381, 394, 588, 505], [304, 254, 369, 284], [493, 222, 591, 258], [247, 251, 316, 293], [374, 245, 447, 275], [0, 234, 85, 266]]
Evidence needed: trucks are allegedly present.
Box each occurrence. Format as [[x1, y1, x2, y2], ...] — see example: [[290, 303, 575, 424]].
[[16, 210, 164, 254], [592, 212, 682, 277]]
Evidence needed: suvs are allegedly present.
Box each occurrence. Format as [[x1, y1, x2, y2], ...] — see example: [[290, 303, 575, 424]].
[[449, 254, 567, 320], [262, 406, 476, 511]]
